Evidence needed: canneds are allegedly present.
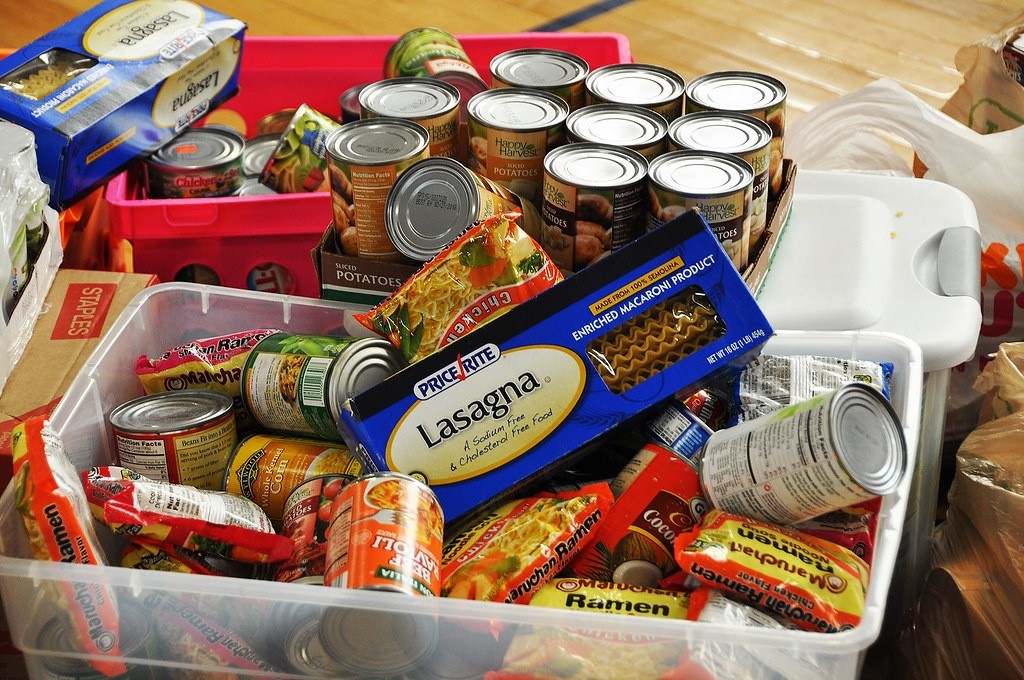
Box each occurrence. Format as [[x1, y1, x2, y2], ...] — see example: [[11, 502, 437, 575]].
[[34, 25, 913, 680]]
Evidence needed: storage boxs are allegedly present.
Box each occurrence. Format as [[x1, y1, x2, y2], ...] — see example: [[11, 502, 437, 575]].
[[751, 167, 989, 619], [0, 281, 924, 680], [102, 31, 634, 299]]
